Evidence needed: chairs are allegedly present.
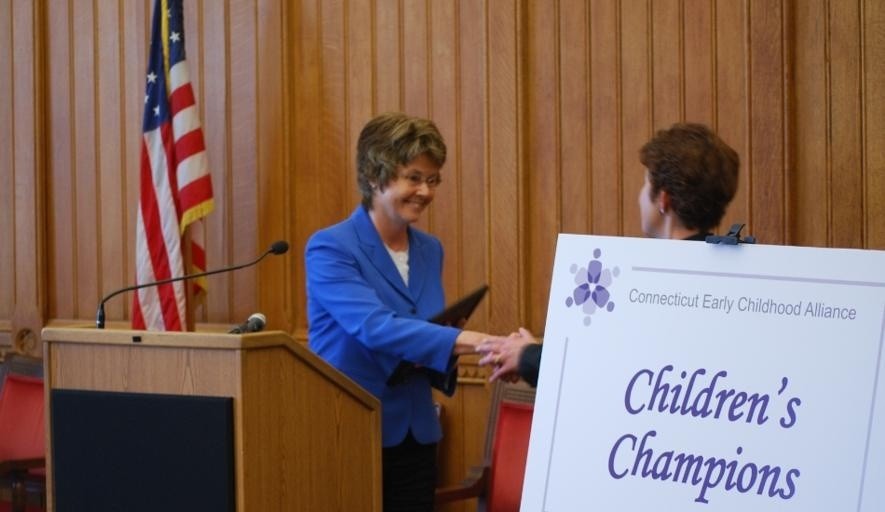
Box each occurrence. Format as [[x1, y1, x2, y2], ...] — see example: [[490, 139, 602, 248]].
[[433, 375, 534, 512], [0, 354, 45, 512]]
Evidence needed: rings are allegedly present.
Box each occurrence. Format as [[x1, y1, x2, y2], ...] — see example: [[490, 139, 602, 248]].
[[495, 355, 501, 365]]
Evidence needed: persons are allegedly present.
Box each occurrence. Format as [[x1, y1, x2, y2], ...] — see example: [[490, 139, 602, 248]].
[[302, 112, 521, 510], [474, 125, 757, 509]]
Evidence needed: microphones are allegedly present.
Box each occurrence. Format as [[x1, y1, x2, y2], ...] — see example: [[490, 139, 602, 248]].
[[96, 240, 289, 329], [227, 312, 266, 333]]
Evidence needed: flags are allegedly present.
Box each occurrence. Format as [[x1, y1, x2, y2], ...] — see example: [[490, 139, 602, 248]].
[[131, 1, 212, 333]]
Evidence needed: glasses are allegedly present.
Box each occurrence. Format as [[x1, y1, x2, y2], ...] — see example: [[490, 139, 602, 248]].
[[402, 171, 441, 188]]
